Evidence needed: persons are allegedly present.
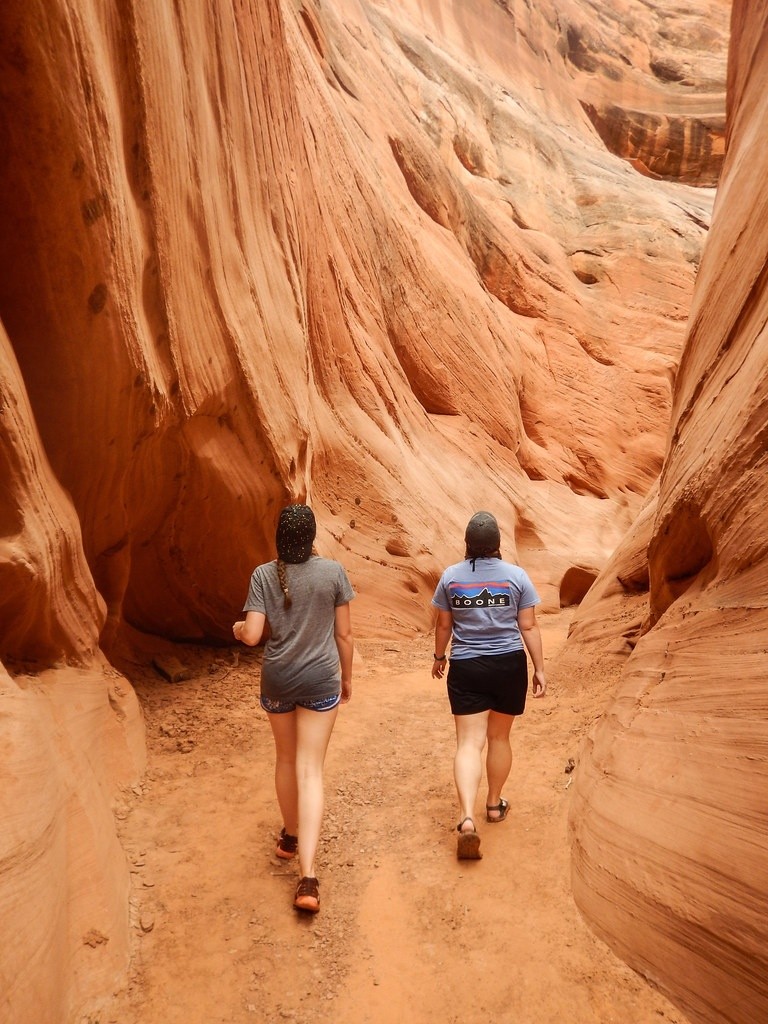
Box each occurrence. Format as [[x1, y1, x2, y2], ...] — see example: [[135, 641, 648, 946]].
[[233, 505, 354, 913], [430, 511, 546, 860]]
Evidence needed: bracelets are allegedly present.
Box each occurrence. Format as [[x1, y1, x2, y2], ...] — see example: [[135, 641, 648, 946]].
[[434, 653, 446, 661]]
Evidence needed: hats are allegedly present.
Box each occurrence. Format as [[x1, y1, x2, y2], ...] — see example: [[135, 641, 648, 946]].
[[466, 511, 500, 554], [275, 504, 316, 563]]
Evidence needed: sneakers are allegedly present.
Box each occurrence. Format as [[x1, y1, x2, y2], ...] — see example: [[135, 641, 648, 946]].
[[276, 828, 298, 858], [295, 877, 321, 912]]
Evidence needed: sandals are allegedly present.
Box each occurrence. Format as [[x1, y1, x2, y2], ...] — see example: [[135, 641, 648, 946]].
[[457, 817, 482, 859], [487, 798, 510, 821]]
